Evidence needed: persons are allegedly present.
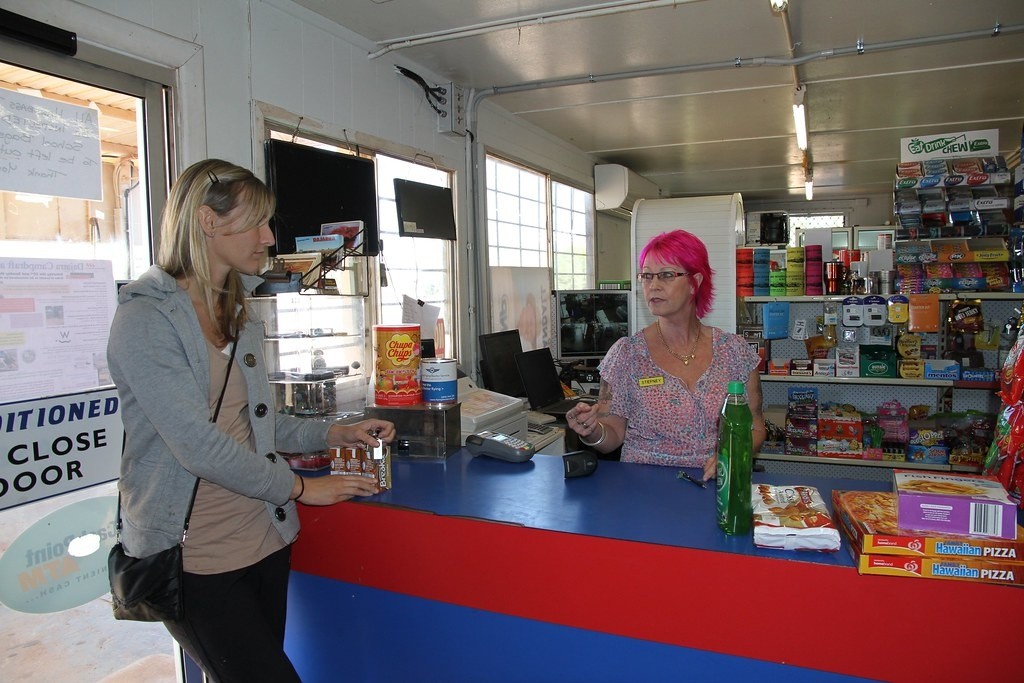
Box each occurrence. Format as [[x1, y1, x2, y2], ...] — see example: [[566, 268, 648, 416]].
[[566, 226, 766, 482], [108, 160, 397, 682]]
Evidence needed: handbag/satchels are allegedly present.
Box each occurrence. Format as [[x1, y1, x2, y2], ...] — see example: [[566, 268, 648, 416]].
[[107, 543, 185, 622]]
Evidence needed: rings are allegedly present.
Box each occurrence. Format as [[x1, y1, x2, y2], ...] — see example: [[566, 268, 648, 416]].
[[587, 427, 593, 432], [568, 414, 572, 419], [583, 423, 588, 428], [575, 418, 582, 425]]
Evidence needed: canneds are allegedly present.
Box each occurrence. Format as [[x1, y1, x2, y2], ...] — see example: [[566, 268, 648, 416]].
[[371, 324, 459, 408]]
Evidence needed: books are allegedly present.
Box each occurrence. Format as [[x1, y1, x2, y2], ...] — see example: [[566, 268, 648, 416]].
[[275, 219, 364, 288]]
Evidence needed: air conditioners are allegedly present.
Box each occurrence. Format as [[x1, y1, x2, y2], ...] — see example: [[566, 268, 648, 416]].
[[594, 164, 671, 221]]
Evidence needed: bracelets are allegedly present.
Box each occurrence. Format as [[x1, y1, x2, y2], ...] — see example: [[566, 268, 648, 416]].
[[579, 421, 606, 446], [294, 474, 305, 499]]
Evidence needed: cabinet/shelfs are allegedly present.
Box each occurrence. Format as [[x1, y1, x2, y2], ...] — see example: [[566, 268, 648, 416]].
[[891, 180, 1017, 292], [743, 292, 1024, 473]]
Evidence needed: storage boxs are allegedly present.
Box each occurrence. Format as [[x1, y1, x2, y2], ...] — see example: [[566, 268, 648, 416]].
[[894, 158, 1011, 287], [831, 469, 1024, 586], [759, 386, 983, 468], [738, 324, 1010, 381]]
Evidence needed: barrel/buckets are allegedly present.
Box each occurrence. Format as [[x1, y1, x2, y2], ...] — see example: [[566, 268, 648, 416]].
[[372, 324, 420, 405], [420, 358, 458, 404]]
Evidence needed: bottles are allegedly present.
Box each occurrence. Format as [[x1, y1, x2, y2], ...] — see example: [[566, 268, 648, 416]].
[[716, 380, 753, 536]]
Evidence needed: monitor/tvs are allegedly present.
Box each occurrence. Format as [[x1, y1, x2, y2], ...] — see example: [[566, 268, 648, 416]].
[[556, 290, 631, 372], [478, 329, 526, 398]]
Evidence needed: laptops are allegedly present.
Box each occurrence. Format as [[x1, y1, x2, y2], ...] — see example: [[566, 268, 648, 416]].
[[515, 347, 597, 416]]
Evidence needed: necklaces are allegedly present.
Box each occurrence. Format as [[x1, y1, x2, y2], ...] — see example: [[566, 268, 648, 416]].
[[656, 318, 701, 365]]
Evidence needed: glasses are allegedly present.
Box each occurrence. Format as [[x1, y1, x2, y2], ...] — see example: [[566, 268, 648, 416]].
[[637, 271, 690, 283]]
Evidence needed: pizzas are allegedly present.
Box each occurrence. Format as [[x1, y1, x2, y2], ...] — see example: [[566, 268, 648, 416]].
[[839, 490, 908, 536]]
